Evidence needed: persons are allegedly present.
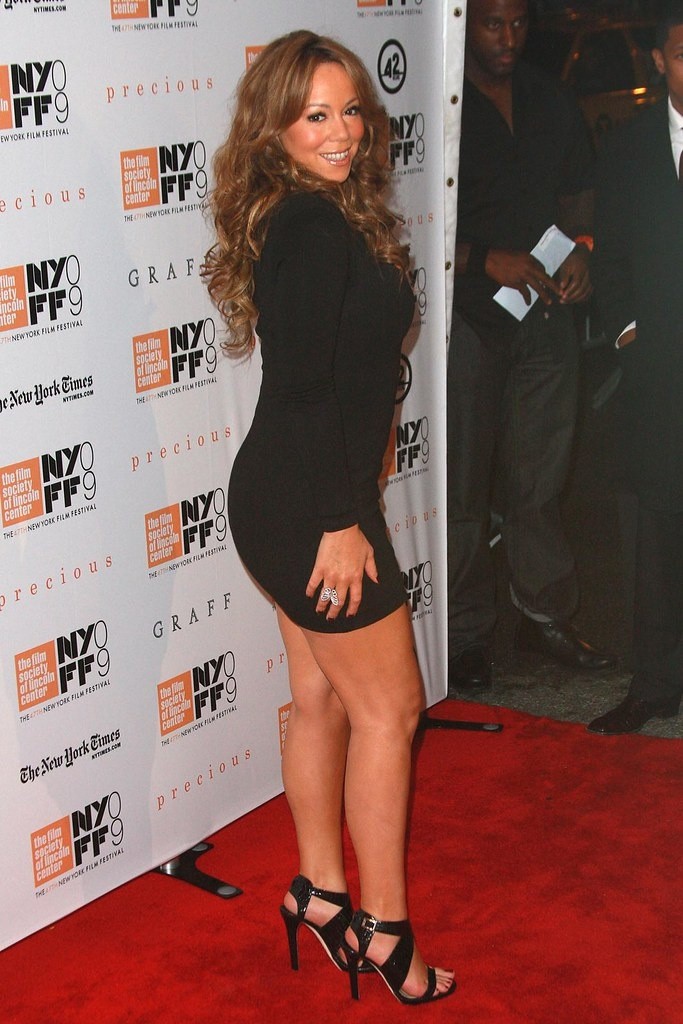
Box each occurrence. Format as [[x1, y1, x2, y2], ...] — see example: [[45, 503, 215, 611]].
[[200, 31, 456, 1006], [448, 0, 683, 736]]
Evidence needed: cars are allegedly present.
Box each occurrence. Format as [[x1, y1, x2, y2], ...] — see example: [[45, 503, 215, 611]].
[[526, 22, 667, 134]]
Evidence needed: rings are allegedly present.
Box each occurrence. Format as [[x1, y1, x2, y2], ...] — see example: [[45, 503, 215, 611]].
[[320, 587, 339, 606]]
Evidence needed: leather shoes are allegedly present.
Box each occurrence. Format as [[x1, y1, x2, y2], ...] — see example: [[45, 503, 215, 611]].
[[448, 658, 492, 695], [585, 694, 679, 734], [470, 643, 539, 688], [499, 606, 623, 680]]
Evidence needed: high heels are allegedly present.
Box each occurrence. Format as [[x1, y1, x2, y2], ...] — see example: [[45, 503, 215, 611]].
[[279, 874, 377, 973], [340, 908, 457, 1005]]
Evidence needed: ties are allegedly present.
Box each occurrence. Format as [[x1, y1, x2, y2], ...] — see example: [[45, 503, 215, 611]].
[[679, 152, 683, 181]]
